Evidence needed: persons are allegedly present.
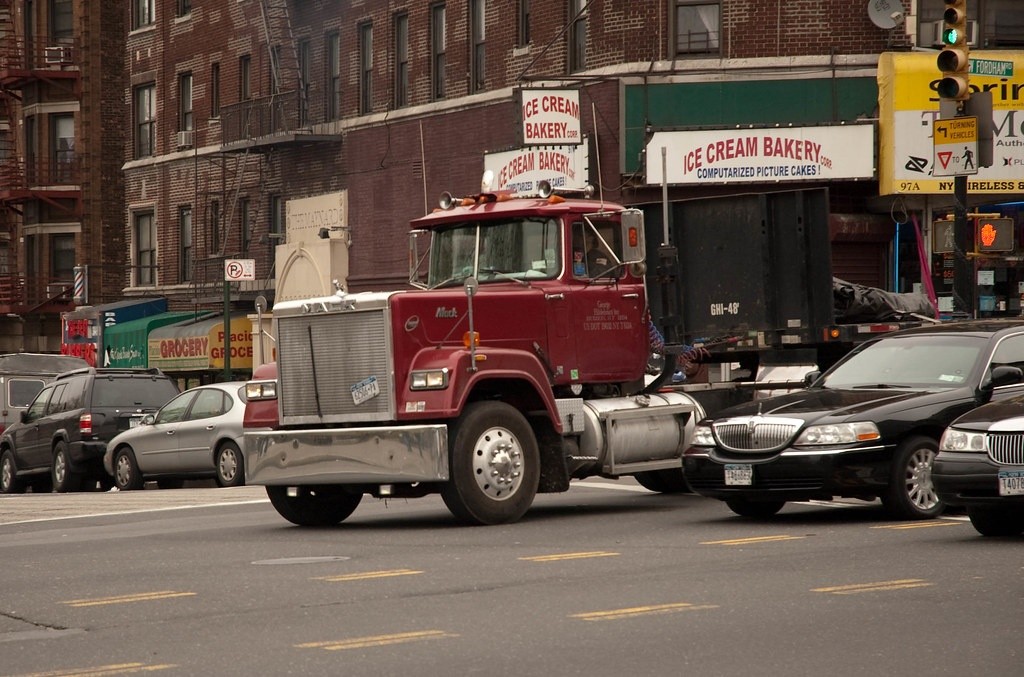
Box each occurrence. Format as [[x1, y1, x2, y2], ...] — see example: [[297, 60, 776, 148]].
[[580, 227, 616, 277]]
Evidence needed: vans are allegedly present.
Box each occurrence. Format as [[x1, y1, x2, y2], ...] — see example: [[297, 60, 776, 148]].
[[1, 351, 93, 436]]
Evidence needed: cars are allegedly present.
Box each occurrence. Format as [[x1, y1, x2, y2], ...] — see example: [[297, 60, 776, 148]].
[[930, 393, 1024, 536], [103, 380, 249, 491], [681, 316, 1023, 521]]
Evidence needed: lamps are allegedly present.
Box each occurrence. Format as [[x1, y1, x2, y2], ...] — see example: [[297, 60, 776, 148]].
[[318, 225, 351, 239]]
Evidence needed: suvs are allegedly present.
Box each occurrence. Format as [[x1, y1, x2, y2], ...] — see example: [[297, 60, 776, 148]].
[[0, 365, 182, 493]]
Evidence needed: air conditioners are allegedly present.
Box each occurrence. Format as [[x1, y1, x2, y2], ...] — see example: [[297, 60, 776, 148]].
[[933, 19, 977, 46], [45, 46, 74, 63], [177, 131, 193, 146]]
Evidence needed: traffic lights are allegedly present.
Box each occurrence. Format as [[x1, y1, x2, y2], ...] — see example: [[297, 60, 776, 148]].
[[977, 217, 1014, 252], [932, 220, 956, 254], [937, 0, 970, 101]]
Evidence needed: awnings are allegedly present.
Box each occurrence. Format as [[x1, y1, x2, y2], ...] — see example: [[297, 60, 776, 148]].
[[61, 296, 258, 370]]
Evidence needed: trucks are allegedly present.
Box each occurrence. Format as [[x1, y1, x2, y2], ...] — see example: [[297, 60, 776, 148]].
[[241, 177, 1024, 529]]
